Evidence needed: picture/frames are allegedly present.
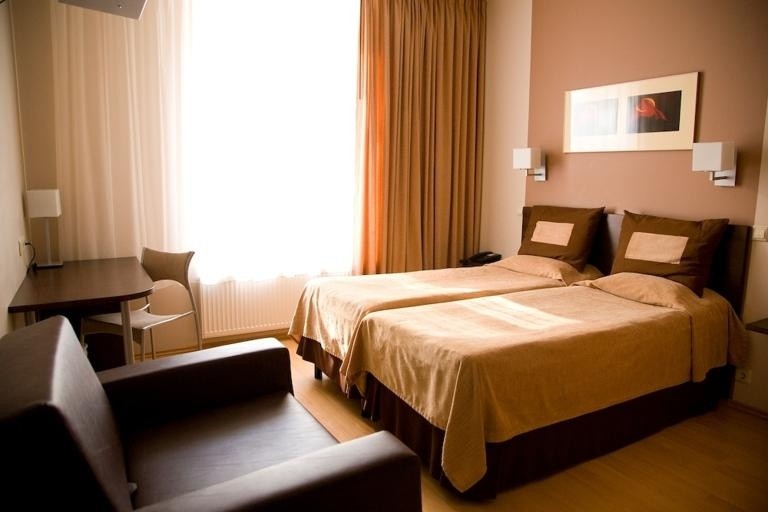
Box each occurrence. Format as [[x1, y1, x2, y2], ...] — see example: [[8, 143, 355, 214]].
[[560, 69, 701, 156]]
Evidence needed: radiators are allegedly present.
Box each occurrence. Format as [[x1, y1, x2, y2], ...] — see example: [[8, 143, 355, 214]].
[[201, 270, 323, 339]]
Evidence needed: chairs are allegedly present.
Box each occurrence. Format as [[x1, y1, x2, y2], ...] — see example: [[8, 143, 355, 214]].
[[1, 312, 423, 512], [78, 247, 205, 366]]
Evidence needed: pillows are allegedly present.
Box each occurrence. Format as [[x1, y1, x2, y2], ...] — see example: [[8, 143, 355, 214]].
[[576, 271, 699, 309], [516, 205, 606, 274], [484, 255, 607, 286], [609, 209, 730, 299]]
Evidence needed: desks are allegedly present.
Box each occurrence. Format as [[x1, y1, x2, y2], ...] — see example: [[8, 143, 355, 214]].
[[9, 254, 156, 366]]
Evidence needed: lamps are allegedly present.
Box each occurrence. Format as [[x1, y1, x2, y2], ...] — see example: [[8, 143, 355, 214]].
[[511, 146, 548, 182], [25, 188, 66, 269], [691, 142, 738, 188]]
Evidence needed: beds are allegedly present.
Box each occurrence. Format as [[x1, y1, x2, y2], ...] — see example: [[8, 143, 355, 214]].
[[286, 207, 607, 420], [343, 211, 755, 500]]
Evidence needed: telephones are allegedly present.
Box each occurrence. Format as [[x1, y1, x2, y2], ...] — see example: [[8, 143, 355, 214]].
[[469, 251, 502, 264]]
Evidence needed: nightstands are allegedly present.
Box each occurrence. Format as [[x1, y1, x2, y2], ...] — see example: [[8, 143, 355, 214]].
[[459, 257, 501, 266], [744, 316, 768, 412]]
